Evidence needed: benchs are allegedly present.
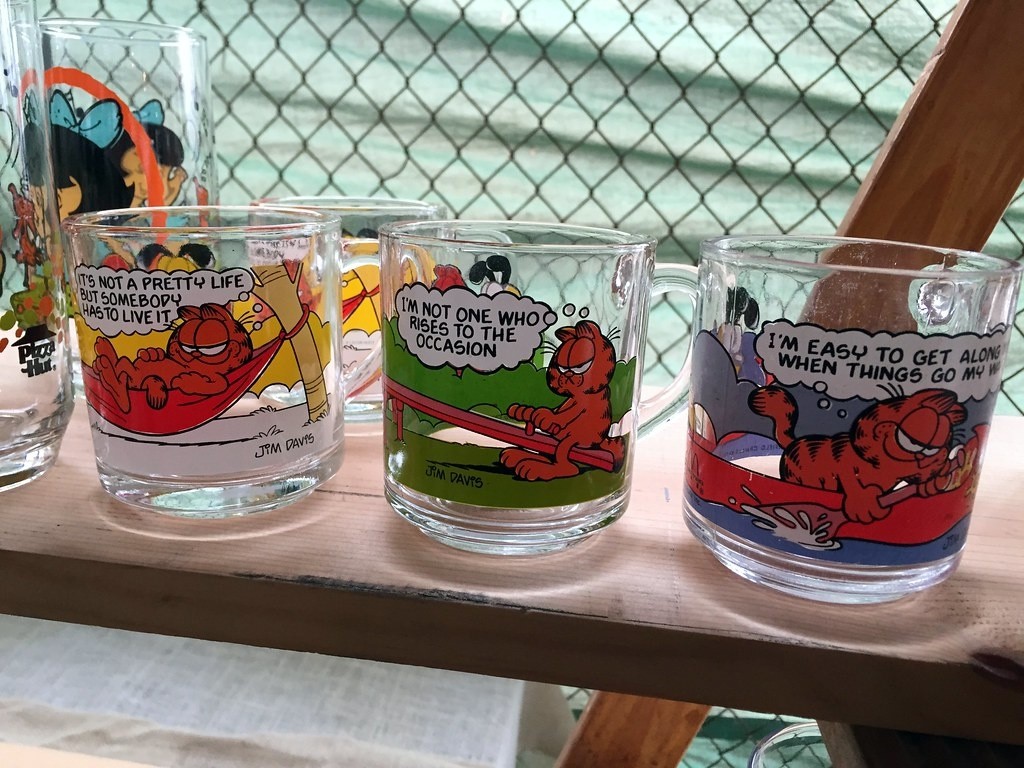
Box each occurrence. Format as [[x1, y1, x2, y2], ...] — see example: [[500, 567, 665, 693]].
[[0, 312, 1024, 768]]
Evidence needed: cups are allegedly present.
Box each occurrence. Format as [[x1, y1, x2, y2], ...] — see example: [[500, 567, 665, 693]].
[[0, 0, 74, 504], [59, 205, 426, 520], [248, 196, 517, 422], [683, 232, 1024, 604], [379, 220, 730, 559], [15, 18, 221, 394]]
